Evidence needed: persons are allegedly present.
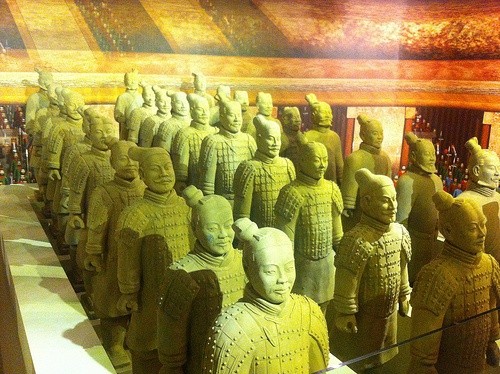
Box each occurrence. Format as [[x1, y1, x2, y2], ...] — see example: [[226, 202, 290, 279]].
[[24, 64, 500, 374], [0, 105, 35, 185]]
